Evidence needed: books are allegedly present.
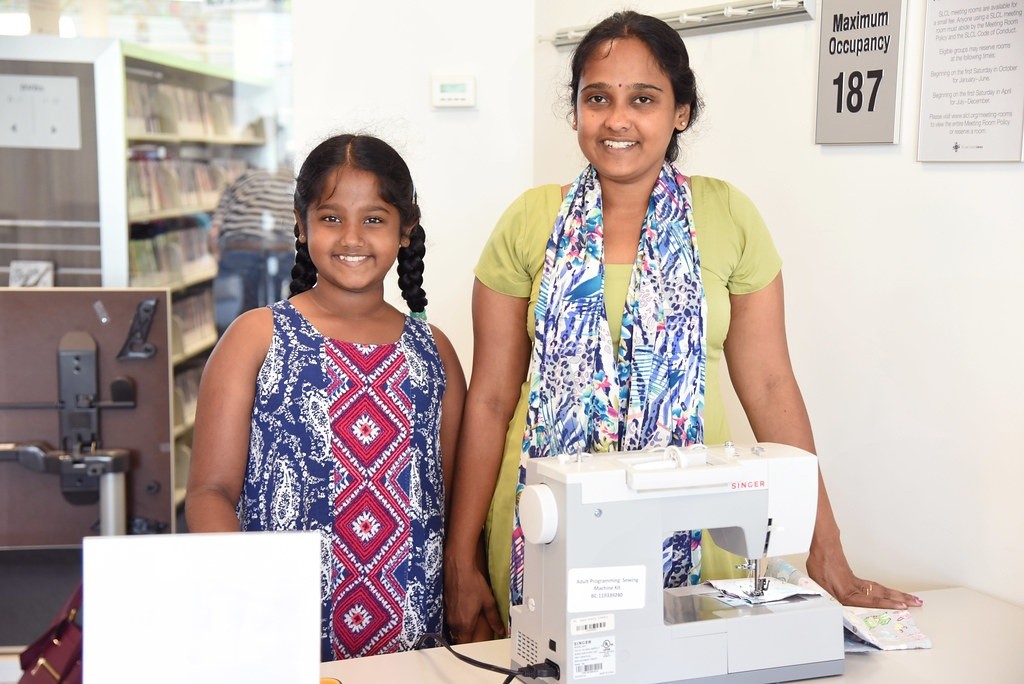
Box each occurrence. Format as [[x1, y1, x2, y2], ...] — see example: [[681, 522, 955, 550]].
[[124, 79, 245, 418]]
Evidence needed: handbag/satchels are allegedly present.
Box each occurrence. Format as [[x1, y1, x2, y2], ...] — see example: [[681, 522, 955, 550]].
[[18, 584, 82, 684]]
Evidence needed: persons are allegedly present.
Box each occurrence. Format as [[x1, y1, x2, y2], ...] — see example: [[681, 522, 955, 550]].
[[185, 134, 495, 663], [208, 171, 297, 339], [444, 12, 922, 644]]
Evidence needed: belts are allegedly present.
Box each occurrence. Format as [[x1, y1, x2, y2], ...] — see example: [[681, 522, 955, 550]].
[[222, 242, 295, 252]]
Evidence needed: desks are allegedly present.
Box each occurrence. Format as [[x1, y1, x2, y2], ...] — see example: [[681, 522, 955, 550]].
[[1, 35, 285, 517], [318, 542, 1024, 684]]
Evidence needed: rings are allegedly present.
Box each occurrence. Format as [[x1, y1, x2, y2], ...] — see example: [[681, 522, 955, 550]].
[[866, 589, 868, 595], [870, 585, 873, 591]]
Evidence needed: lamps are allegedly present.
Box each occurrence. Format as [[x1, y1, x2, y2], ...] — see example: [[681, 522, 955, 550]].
[[553, 0, 814, 52]]
